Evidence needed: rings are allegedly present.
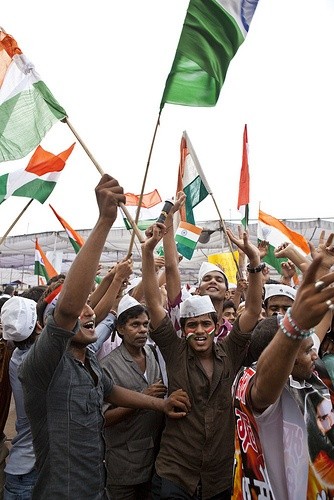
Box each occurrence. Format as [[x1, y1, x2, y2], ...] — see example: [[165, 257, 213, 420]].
[[326, 300, 334, 308], [127, 259, 131, 264], [315, 279, 324, 290]]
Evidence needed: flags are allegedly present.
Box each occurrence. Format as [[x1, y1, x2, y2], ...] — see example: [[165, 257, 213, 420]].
[[0, 0, 311, 285]]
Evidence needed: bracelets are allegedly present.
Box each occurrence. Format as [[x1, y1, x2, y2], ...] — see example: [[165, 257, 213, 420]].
[[279, 307, 315, 340], [246, 263, 265, 272]]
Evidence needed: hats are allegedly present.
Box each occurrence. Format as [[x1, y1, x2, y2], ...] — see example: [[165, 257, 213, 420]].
[[0, 294, 11, 299], [181, 285, 192, 301], [188, 287, 196, 294], [116, 293, 142, 320], [309, 333, 321, 355], [197, 261, 229, 291], [263, 283, 298, 302], [122, 274, 142, 295], [227, 283, 237, 289], [1, 296, 38, 342], [179, 294, 217, 318]]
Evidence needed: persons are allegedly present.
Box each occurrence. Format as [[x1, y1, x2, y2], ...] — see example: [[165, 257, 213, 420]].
[[18, 174, 192, 500], [0, 191, 334, 500], [306, 390, 334, 463]]
